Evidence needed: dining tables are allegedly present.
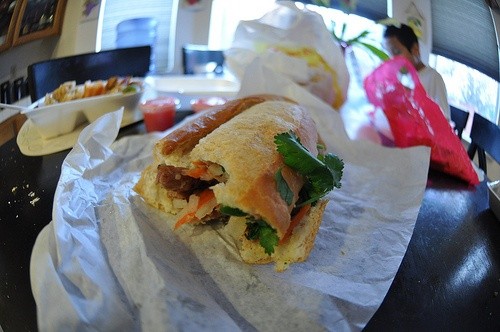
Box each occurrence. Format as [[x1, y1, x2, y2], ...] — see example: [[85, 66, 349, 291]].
[[0, 91, 499, 332]]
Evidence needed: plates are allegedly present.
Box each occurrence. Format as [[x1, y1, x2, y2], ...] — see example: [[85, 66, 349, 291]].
[[145, 75, 240, 98]]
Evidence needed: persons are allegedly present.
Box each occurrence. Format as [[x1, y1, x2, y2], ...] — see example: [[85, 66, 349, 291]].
[[374, 20, 452, 140]]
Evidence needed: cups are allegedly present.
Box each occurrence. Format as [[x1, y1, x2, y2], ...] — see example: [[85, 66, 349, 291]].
[[138, 96, 178, 132]]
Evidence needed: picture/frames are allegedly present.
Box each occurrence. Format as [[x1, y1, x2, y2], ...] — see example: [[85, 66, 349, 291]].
[[0, 0, 68, 52]]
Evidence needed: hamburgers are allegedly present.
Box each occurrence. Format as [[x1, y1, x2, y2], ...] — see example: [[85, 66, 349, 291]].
[[131, 92, 345, 273]]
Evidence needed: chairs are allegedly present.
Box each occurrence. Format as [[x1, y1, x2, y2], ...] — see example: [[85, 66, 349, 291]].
[[182, 45, 224, 76], [27, 46, 151, 104], [450, 105, 500, 175]]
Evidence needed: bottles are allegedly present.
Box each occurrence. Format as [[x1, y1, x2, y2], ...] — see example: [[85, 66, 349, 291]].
[[20, 3, 56, 35], [114, 16, 159, 73]]
